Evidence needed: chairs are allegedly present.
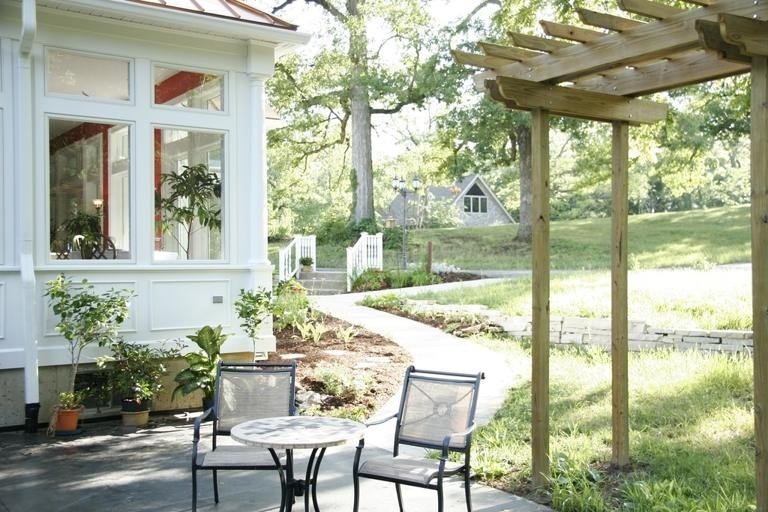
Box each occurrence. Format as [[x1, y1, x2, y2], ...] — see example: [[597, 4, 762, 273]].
[[353, 366, 485, 512], [192, 360, 298, 512]]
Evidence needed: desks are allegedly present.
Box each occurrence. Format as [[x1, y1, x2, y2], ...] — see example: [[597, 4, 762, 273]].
[[231, 416, 367, 511]]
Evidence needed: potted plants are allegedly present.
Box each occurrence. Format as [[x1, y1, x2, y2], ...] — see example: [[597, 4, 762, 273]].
[[171, 325, 236, 421], [42, 272, 136, 432], [104, 340, 188, 427]]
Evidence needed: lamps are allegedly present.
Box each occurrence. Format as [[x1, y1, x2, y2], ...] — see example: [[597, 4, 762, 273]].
[[93, 199, 104, 216]]
